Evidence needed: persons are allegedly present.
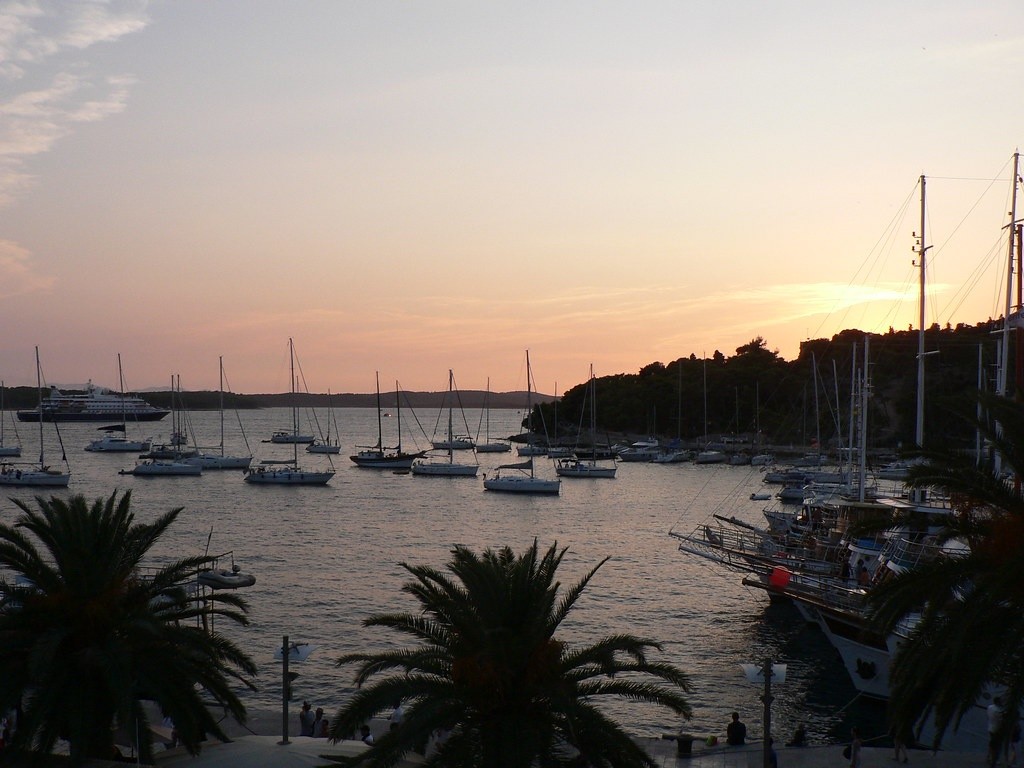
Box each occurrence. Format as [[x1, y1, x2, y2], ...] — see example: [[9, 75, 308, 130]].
[[135, 457, 157, 465], [726, 711, 746, 745], [856, 558, 869, 585], [300, 701, 330, 738], [850, 725, 863, 768], [837, 556, 853, 586], [389, 452, 410, 457], [785, 529, 796, 536], [648, 434, 655, 439], [889, 710, 909, 763], [986, 696, 1020, 766], [11, 469, 14, 471], [791, 722, 807, 744], [413, 727, 449, 757], [360, 725, 375, 742], [158, 443, 166, 451], [257, 467, 265, 473], [16, 469, 22, 479], [1, 463, 7, 473], [44, 466, 47, 470], [276, 432, 281, 436], [386, 701, 404, 729], [310, 439, 316, 446], [770, 738, 778, 768]]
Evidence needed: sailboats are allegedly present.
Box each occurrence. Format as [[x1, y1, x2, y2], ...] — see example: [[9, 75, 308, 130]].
[[0, 379, 23, 457], [241, 335, 338, 484], [430, 368, 478, 450], [411, 373, 481, 476], [514, 384, 551, 458], [348, 370, 430, 469], [270, 376, 316, 445], [545, 358, 659, 477], [0, 345, 73, 485], [480, 349, 562, 496], [303, 389, 342, 454], [694, 148, 1023, 748], [473, 375, 513, 452], [83, 352, 255, 476], [650, 359, 693, 463]]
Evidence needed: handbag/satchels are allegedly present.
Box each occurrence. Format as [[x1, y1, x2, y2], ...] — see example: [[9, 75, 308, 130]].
[[843, 744, 851, 759], [707, 736, 717, 746]]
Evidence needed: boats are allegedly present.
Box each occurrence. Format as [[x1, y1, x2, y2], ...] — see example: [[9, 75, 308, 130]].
[[16, 379, 172, 421]]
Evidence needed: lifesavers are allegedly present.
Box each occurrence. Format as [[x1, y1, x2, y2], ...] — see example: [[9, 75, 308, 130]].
[[250, 469, 255, 476], [258, 469, 264, 473], [848, 496, 860, 501], [923, 547, 934, 557]]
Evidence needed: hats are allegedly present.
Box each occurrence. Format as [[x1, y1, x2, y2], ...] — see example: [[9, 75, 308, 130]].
[[315, 707, 326, 714], [360, 725, 370, 731], [302, 700, 311, 708]]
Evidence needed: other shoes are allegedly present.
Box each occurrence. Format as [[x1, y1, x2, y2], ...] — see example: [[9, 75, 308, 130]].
[[901, 757, 909, 763], [892, 757, 899, 761]]
